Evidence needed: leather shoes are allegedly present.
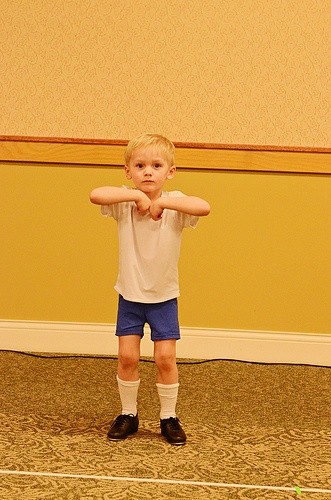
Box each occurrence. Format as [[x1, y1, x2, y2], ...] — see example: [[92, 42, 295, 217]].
[[107, 412, 139, 440], [160, 417, 187, 446]]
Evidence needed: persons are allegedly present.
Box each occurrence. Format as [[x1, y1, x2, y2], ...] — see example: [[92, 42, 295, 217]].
[[90, 134, 212, 445]]
